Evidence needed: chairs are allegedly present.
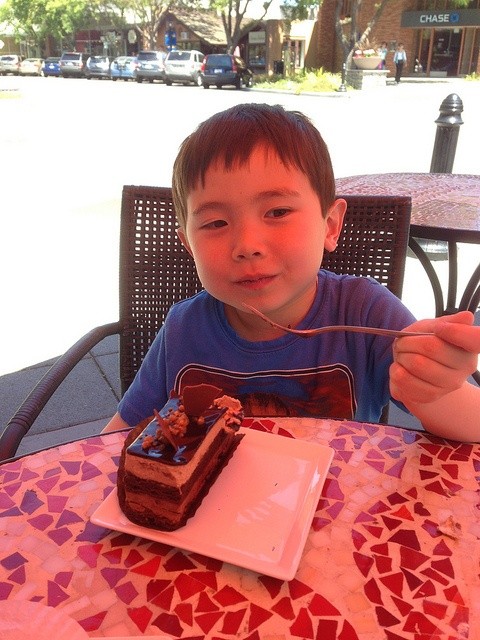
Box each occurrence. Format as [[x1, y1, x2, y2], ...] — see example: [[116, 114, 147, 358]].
[[1, 185, 414, 462]]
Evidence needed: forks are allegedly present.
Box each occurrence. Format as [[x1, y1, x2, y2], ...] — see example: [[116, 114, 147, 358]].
[[243, 302, 435, 339]]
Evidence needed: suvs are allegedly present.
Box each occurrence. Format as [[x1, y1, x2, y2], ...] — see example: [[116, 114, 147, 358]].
[[164, 50, 202, 85], [59, 51, 83, 78], [134, 51, 165, 83], [200, 54, 251, 89], [0, 55, 21, 77]]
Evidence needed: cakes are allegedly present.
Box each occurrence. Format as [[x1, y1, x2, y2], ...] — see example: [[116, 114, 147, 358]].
[[116, 384, 244, 530]]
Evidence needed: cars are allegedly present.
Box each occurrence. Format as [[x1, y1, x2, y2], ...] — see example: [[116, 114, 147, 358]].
[[42, 57, 62, 78], [20, 57, 44, 77], [110, 56, 135, 82], [84, 55, 109, 79]]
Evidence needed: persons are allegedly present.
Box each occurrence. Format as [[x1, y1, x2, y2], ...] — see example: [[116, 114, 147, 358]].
[[376, 41, 388, 69], [99, 103, 480, 443], [393, 43, 407, 82]]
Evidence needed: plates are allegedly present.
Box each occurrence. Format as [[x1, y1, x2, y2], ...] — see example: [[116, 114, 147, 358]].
[[89, 425, 336, 581]]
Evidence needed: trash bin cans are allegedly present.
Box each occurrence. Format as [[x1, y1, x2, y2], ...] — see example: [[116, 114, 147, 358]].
[[274, 60, 284, 77]]
